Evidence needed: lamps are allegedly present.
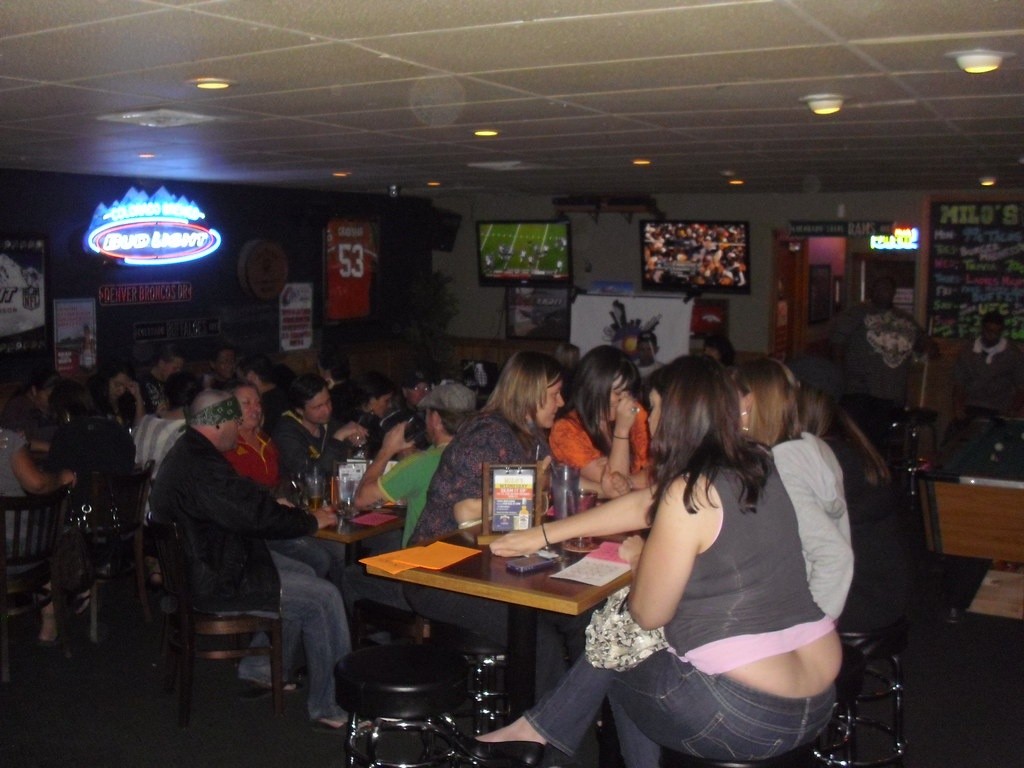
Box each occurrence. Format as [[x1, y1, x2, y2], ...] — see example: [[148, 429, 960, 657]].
[[800, 93, 851, 114], [944, 48, 1014, 74]]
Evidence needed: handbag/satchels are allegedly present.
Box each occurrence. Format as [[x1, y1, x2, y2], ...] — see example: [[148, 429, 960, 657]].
[[583, 585, 666, 670], [64, 493, 122, 534]]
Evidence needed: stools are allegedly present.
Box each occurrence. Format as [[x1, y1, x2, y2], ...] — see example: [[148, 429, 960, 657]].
[[333, 643, 489, 768], [659, 712, 849, 768], [456, 647, 509, 737], [812, 614, 911, 768]]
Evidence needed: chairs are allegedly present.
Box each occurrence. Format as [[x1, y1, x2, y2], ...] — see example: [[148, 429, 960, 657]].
[[145, 507, 287, 728], [70, 458, 155, 642], [0, 483, 68, 686], [871, 403, 943, 564]]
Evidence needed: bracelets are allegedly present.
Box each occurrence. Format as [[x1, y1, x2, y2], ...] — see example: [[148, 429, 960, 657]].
[[612, 434, 630, 440], [541, 521, 552, 547]]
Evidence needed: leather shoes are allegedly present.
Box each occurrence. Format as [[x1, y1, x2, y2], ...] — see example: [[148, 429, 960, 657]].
[[447, 732, 547, 768]]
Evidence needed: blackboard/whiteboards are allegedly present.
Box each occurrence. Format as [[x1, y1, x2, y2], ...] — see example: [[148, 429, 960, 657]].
[[918, 191, 1024, 355]]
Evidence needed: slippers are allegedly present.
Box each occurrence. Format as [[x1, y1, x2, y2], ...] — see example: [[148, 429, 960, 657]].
[[246, 680, 298, 693], [311, 713, 373, 732]]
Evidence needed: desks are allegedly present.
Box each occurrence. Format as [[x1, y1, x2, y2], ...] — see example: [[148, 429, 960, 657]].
[[356, 494, 641, 726], [914, 410, 1024, 624], [297, 500, 407, 563]]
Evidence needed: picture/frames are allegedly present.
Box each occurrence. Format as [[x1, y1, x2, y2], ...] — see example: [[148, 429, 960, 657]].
[[833, 273, 850, 312], [809, 259, 831, 323], [477, 460, 543, 546]]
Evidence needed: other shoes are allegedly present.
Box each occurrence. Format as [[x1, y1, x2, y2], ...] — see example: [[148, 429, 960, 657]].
[[72, 587, 94, 614], [94, 569, 110, 583]]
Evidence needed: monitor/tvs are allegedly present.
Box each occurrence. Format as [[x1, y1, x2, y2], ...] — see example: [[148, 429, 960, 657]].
[[475, 219, 572, 285], [639, 221, 751, 292]]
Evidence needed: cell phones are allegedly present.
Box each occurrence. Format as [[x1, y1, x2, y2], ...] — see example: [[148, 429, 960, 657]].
[[507, 554, 561, 573]]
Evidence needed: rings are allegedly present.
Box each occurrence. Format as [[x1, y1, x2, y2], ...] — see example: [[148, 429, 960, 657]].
[[631, 407, 640, 414]]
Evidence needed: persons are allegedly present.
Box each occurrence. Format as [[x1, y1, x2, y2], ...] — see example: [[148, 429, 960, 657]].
[[830, 276, 941, 413], [948, 309, 1024, 429], [484, 236, 568, 274], [146, 389, 381, 738], [780, 356, 913, 633], [0, 330, 746, 651], [643, 223, 747, 288], [402, 351, 630, 768], [729, 355, 855, 632], [543, 309, 568, 337], [446, 352, 845, 768]]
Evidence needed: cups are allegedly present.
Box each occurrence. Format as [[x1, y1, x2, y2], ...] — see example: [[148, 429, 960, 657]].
[[553, 464, 579, 520], [331, 477, 355, 515], [305, 475, 325, 511], [404, 416, 427, 442]]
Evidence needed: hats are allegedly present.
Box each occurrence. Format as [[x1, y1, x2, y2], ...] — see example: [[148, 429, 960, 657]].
[[417, 383, 476, 410]]
